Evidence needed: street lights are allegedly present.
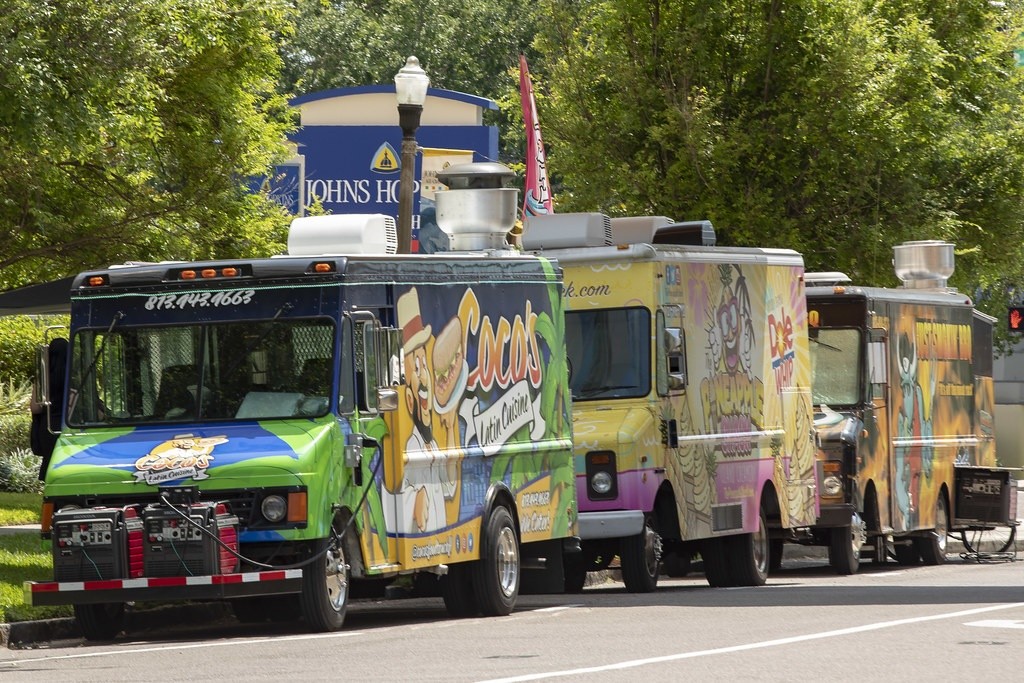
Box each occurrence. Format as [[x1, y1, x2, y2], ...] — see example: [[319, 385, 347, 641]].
[[394, 55, 431, 254]]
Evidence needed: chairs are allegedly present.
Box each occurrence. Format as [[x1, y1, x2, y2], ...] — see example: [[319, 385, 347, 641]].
[[153, 363, 206, 416], [293, 357, 333, 401]]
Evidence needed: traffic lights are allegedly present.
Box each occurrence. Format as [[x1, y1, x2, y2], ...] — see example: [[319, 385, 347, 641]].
[[1008, 306, 1024, 332]]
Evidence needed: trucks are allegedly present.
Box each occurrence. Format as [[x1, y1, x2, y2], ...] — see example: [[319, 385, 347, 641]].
[[22, 162, 999, 641]]
